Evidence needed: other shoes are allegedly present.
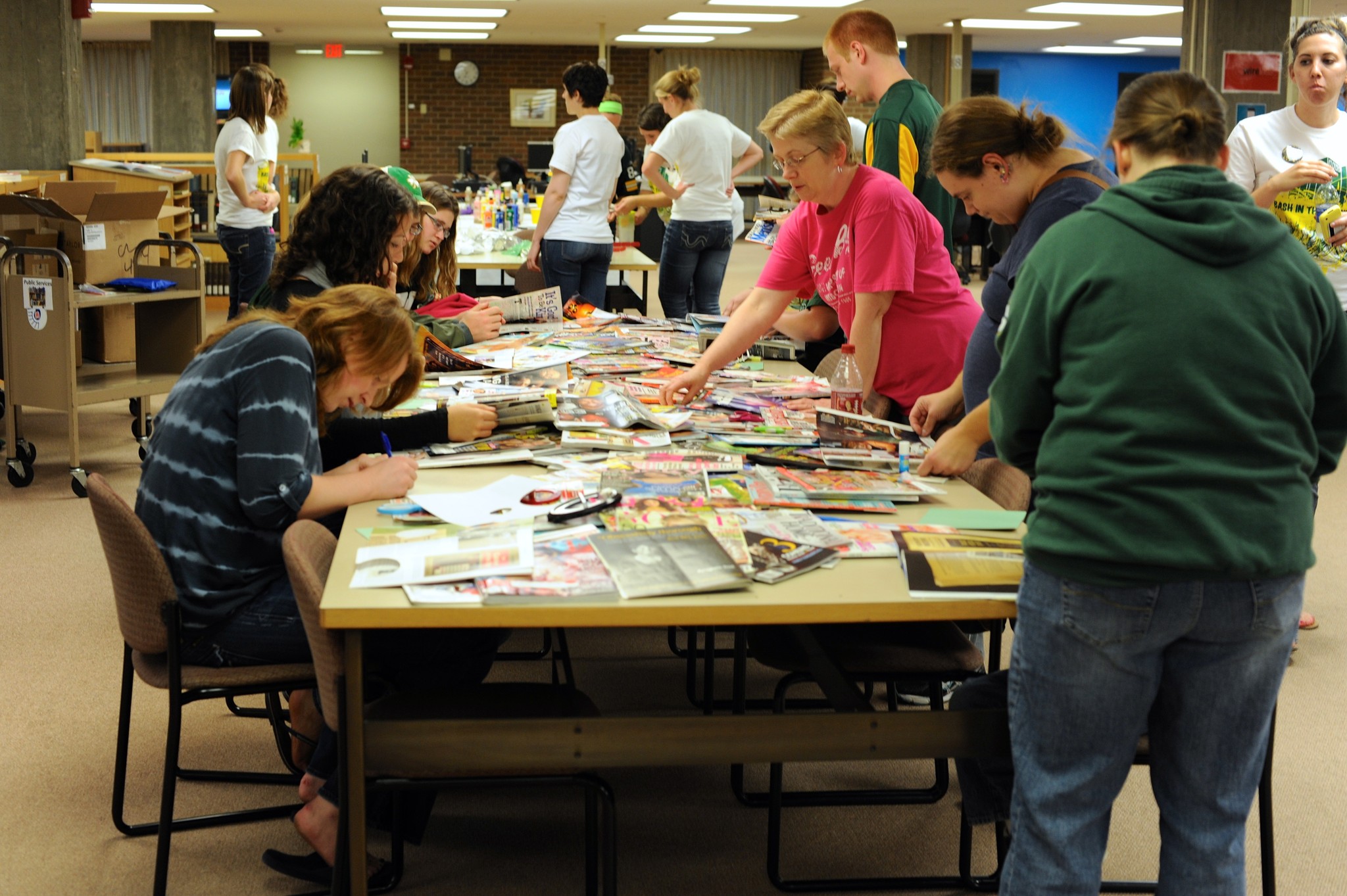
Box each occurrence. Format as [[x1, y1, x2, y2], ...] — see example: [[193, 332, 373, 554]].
[[896, 676, 961, 706]]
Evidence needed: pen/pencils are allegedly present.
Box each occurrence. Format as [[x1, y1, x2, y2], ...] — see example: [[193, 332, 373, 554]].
[[754, 426, 785, 434], [381, 431, 392, 457]]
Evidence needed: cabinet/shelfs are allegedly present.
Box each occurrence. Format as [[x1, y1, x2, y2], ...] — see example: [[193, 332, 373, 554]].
[[69, 158, 193, 273]]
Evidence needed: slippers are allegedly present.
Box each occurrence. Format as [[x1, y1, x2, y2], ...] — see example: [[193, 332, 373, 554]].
[[267, 686, 317, 777], [262, 846, 393, 890]]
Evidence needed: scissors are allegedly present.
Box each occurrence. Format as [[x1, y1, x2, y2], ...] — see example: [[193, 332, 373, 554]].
[[711, 410, 765, 423]]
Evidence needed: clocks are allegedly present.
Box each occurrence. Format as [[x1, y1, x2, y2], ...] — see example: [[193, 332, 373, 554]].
[[454, 61, 479, 86]]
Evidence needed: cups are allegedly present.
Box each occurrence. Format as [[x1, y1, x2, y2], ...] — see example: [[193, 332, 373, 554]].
[[530, 208, 540, 224], [535, 194, 545, 209], [618, 210, 635, 243]]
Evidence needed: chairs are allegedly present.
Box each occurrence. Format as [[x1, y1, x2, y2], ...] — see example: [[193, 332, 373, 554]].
[[83, 471, 402, 896]]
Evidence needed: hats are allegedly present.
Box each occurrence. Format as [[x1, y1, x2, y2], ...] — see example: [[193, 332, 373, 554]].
[[381, 166, 437, 215]]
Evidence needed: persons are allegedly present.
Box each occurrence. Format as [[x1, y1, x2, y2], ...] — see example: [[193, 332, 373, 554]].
[[497, 157, 526, 188], [910, 96, 1118, 476], [822, 10, 958, 265], [816, 78, 847, 106], [722, 286, 844, 374], [249, 166, 498, 771], [135, 282, 426, 895], [395, 182, 502, 310], [614, 102, 745, 313], [215, 63, 281, 321], [600, 89, 665, 261], [526, 61, 625, 311], [1224, 18, 1347, 652], [642, 66, 764, 320], [659, 89, 986, 705], [382, 165, 506, 349], [257, 79, 289, 227], [990, 71, 1347, 896]]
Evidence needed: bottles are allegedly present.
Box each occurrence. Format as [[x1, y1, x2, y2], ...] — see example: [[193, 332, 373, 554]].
[[516, 178, 525, 192], [504, 182, 524, 230], [489, 184, 504, 230], [465, 186, 472, 203], [831, 344, 864, 416], [1315, 175, 1339, 238], [473, 190, 492, 228]]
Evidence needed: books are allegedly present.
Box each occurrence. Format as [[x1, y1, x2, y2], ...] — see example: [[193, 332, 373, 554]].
[[350, 289, 1028, 605]]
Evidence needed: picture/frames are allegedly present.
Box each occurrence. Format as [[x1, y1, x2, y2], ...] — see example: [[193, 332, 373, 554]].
[[510, 88, 557, 127]]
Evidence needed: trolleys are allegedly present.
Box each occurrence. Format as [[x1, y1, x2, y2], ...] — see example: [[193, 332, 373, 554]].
[[1, 237, 208, 498]]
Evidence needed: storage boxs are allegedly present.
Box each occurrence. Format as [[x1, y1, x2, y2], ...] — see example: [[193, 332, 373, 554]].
[[0, 182, 167, 369]]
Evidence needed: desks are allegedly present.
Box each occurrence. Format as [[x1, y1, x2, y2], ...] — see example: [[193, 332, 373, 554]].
[[455, 204, 658, 316], [320, 324, 1023, 896]]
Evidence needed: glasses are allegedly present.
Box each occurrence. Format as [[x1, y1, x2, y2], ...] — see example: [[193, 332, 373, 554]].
[[773, 146, 821, 171], [425, 212, 450, 238], [409, 223, 423, 236]]
